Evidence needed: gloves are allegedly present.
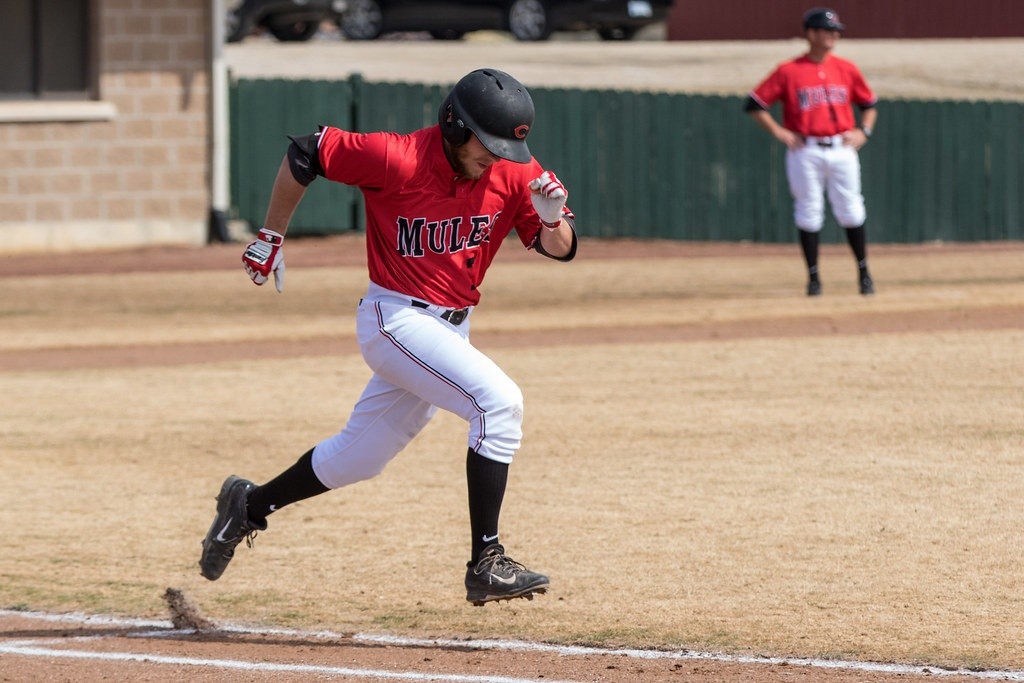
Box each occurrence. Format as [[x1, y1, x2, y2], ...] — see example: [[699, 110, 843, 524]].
[[527, 170, 569, 232], [241, 227, 286, 294]]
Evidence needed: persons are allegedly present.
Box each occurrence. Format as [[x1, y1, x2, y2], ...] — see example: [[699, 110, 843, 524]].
[[198, 68, 577, 604], [743, 7, 879, 296]]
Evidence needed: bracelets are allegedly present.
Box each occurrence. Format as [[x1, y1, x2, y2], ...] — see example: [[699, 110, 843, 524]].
[[861, 125, 873, 138]]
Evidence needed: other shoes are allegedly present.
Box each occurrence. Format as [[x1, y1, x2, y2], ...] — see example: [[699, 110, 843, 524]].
[[807, 280, 821, 296], [860, 274, 874, 295]]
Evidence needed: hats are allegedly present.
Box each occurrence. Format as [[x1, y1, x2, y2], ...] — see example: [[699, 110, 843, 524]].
[[802, 6, 845, 31]]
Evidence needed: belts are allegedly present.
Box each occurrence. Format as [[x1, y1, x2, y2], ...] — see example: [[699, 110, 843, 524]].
[[412, 299, 469, 325], [801, 137, 838, 147]]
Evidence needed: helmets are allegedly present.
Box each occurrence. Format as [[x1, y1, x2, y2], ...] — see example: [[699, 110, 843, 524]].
[[449, 68, 535, 164]]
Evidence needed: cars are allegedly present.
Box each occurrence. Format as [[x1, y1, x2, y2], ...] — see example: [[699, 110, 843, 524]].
[[223, 0, 678, 42]]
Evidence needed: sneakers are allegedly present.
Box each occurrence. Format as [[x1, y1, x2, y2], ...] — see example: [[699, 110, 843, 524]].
[[465, 543, 550, 607], [198, 474, 267, 582]]
